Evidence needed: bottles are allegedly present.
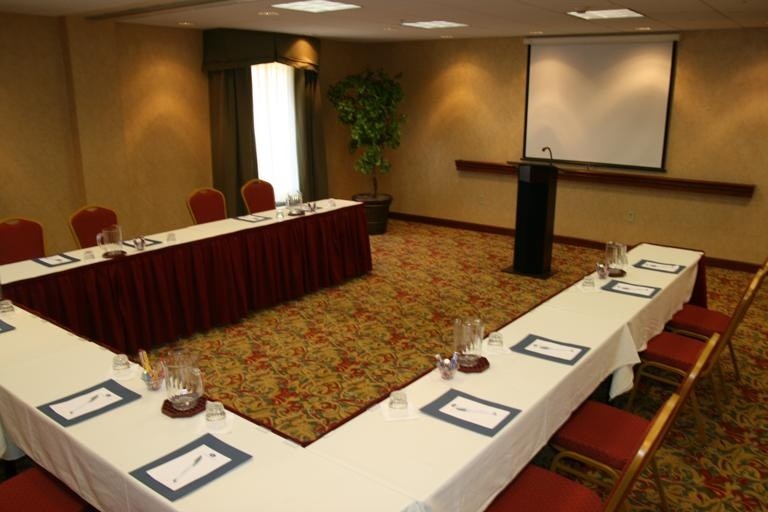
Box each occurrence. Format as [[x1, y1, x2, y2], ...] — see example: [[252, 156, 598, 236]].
[[134, 237, 145, 251]]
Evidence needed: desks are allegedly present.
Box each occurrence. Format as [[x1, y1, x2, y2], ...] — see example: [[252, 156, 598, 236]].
[[0, 198, 708, 512]]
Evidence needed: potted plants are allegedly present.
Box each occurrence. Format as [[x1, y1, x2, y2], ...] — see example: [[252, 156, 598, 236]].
[[327, 69, 409, 232]]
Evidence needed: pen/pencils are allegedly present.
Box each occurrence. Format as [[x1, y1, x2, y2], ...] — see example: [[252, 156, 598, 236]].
[[70, 394, 98, 415], [173, 456, 203, 483], [533, 344, 574, 353], [454, 407, 497, 417], [140, 349, 166, 391], [431, 351, 459, 380]]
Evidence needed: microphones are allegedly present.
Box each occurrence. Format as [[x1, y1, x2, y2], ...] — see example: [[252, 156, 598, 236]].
[[541, 146, 552, 166]]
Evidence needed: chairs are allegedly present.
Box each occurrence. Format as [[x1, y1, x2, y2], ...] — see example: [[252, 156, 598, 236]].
[[627, 261, 767, 442], [550, 334, 722, 502], [1, 217, 45, 262], [0, 464, 89, 512], [240, 179, 278, 212], [66, 205, 119, 249], [185, 188, 226, 224], [490, 393, 682, 510]]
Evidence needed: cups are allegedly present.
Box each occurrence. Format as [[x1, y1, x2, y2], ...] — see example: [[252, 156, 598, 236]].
[[112, 353, 131, 371], [583, 240, 628, 288], [437, 316, 484, 381], [0, 299, 15, 313], [162, 346, 203, 412], [96, 225, 123, 256], [275, 191, 337, 220], [166, 232, 177, 242], [82, 251, 95, 260], [487, 332, 503, 348], [141, 372, 165, 390], [205, 401, 226, 422], [389, 390, 409, 409]]
